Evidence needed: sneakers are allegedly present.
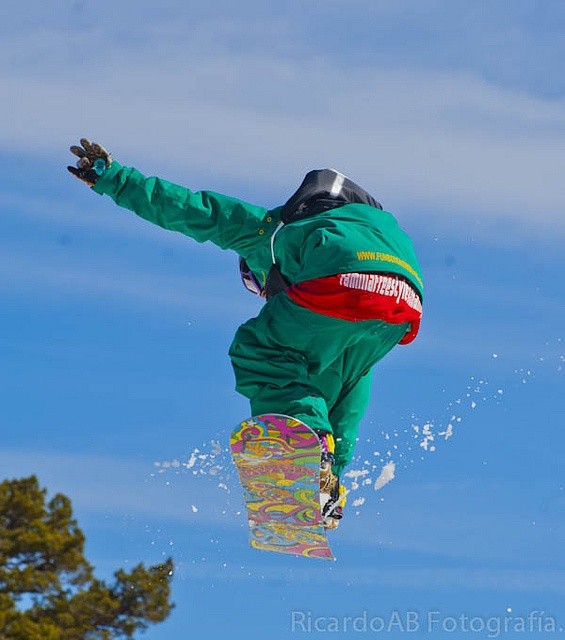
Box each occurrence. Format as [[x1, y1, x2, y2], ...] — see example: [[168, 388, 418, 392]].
[[322, 498, 341, 529], [318, 453, 335, 492]]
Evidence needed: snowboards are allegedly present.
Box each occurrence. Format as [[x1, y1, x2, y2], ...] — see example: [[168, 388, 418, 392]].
[[230, 414, 335, 560]]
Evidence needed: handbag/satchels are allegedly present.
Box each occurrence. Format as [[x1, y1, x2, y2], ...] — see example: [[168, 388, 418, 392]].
[[280, 167, 383, 225]]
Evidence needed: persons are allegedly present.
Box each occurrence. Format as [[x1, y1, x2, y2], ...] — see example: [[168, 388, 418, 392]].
[[65, 137, 424, 530]]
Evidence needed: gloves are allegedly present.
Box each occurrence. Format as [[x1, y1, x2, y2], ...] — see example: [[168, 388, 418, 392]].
[[67, 137, 111, 187]]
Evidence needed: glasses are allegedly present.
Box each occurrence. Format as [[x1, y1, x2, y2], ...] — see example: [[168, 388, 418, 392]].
[[242, 271, 260, 294]]
